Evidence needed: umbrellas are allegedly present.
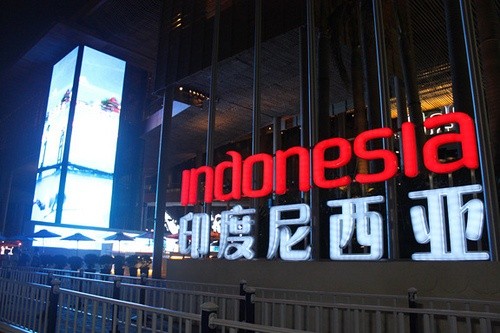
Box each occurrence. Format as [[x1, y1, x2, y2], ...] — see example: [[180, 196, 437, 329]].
[[0, 234, 35, 256], [61, 233, 95, 255], [27, 230, 61, 256], [168, 233, 191, 259], [135, 231, 166, 248], [105, 232, 134, 257]]
